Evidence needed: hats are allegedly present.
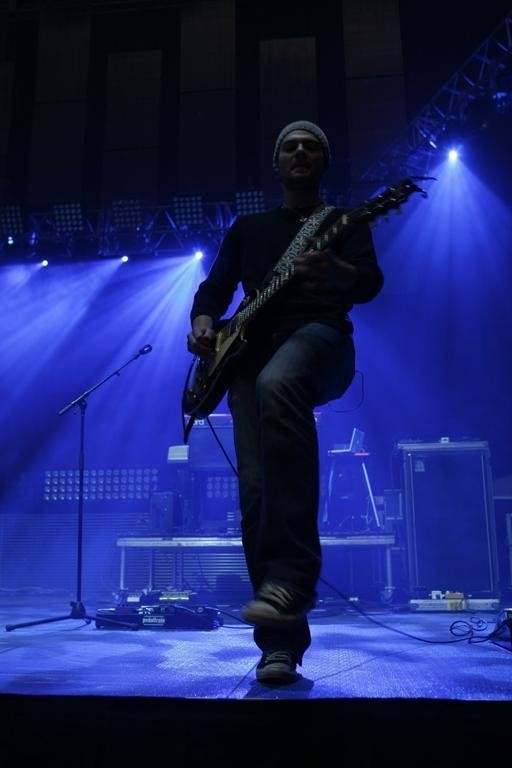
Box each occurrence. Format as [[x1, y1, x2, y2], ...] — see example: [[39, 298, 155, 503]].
[[271, 121, 331, 176]]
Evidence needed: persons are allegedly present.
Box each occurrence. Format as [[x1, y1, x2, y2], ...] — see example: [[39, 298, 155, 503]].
[[186, 120, 382, 685]]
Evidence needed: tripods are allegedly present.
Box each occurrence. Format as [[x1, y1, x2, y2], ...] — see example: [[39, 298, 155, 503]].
[[6, 407, 142, 631]]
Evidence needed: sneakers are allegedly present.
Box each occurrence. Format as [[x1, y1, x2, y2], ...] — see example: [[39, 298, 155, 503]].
[[245, 581, 319, 621], [255, 651, 299, 679]]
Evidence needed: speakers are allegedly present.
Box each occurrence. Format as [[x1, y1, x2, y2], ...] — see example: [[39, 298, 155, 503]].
[[395, 433, 502, 600]]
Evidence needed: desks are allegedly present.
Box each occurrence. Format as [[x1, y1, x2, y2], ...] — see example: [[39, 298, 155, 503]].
[[115, 534, 398, 603]]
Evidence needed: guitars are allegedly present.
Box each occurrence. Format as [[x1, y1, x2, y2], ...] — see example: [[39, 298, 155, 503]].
[[183, 177, 422, 420]]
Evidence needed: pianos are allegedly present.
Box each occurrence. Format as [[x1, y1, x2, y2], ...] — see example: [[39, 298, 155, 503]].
[[182, 411, 324, 430]]
